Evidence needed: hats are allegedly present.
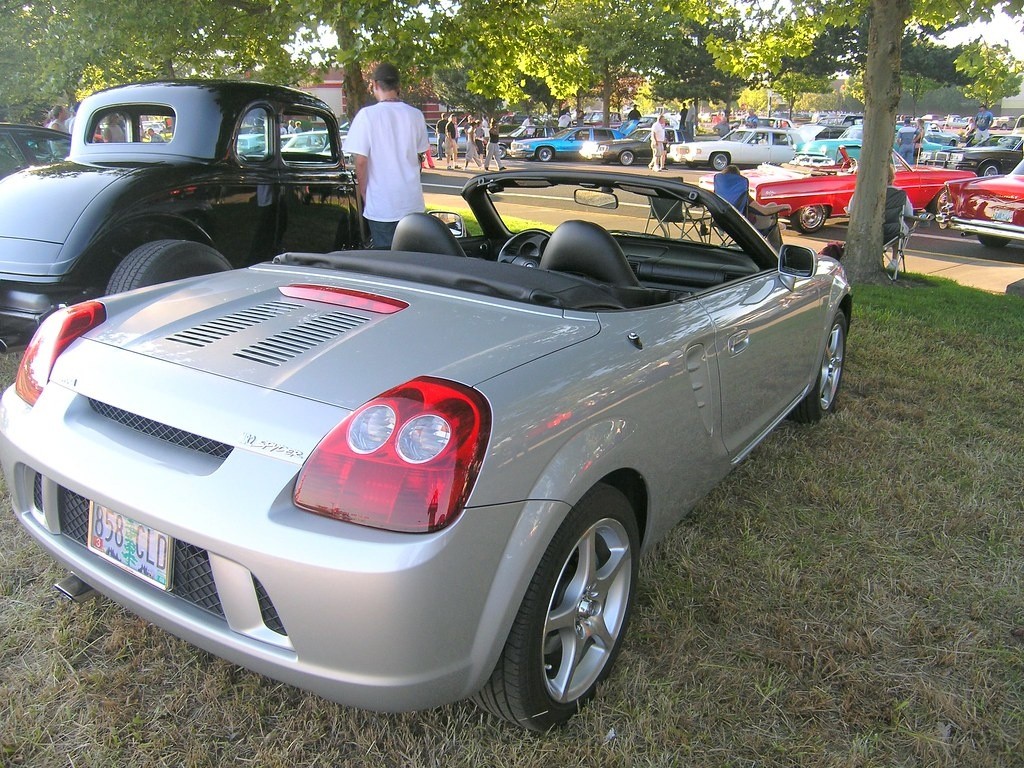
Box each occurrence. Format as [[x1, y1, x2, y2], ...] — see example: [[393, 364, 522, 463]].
[[979, 104, 986, 108], [372, 63, 401, 80]]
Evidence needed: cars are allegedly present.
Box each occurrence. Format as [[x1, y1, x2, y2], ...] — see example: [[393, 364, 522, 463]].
[[926, 134, 1023, 178], [897, 121, 961, 147], [0, 75, 370, 356], [0, 122, 75, 176], [694, 111, 1024, 159], [667, 124, 832, 170], [482, 110, 697, 166], [804, 124, 956, 175], [238, 116, 491, 163]]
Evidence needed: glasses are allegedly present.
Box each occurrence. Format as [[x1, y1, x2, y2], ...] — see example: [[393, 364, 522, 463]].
[[661, 124, 665, 129]]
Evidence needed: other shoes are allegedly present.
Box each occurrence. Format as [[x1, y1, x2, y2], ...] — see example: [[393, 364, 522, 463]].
[[447, 166, 454, 170], [886, 259, 900, 271], [455, 165, 461, 169], [778, 222, 786, 231], [437, 158, 442, 160], [654, 167, 661, 172], [660, 167, 668, 171], [648, 163, 653, 170]]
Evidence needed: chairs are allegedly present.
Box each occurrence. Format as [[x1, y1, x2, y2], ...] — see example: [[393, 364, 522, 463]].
[[535, 220, 644, 287], [843, 188, 933, 284], [926, 152, 951, 168], [392, 213, 466, 258], [710, 174, 789, 247], [644, 176, 713, 243]]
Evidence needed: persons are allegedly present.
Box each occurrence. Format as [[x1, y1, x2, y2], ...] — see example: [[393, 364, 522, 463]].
[[421, 101, 758, 173], [43, 105, 302, 147], [342, 64, 428, 247], [257, 183, 287, 258], [723, 105, 995, 271]]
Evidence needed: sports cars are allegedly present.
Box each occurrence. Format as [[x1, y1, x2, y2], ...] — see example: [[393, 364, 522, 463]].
[[937, 157, 1024, 250], [696, 141, 979, 235], [0, 163, 859, 736]]
